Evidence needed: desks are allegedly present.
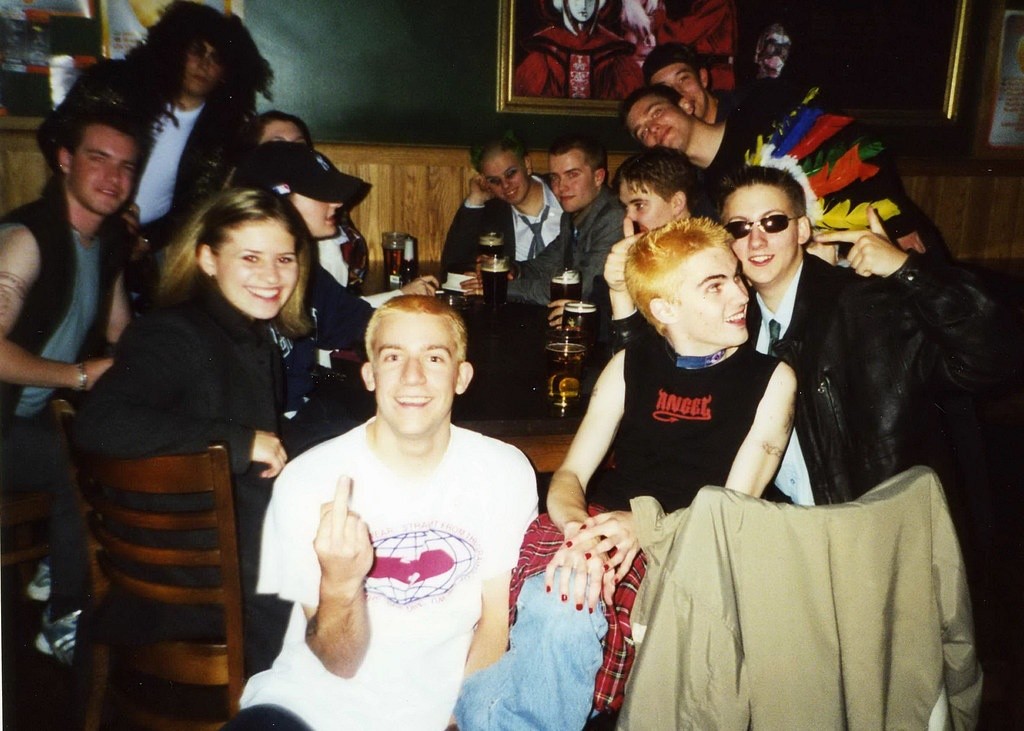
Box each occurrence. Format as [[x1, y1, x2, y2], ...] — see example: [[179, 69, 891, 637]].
[[315, 322, 618, 477]]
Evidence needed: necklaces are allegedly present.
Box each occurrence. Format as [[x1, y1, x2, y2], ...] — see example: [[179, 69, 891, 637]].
[[435, 135, 564, 287]]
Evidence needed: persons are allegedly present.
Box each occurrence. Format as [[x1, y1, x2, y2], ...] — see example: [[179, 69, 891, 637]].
[[602, 167, 1007, 507], [463, 135, 621, 308], [456, 220, 796, 731], [220, 293, 539, 731], [35, 0, 273, 272], [0, 101, 131, 667], [549, 146, 715, 332], [220, 107, 440, 455], [74, 187, 319, 674], [614, 44, 956, 279]]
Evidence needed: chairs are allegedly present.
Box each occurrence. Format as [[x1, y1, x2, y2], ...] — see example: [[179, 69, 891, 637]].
[[607, 464, 981, 731], [1, 401, 250, 731]]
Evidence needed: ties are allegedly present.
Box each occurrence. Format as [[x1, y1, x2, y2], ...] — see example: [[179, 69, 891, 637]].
[[767, 319, 781, 358], [519, 206, 550, 262]]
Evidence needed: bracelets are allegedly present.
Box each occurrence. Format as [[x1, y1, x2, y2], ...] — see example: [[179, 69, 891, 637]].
[[78, 363, 88, 392], [143, 238, 149, 244]]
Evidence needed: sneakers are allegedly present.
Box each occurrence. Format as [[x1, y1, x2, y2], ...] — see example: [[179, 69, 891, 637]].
[[35, 607, 80, 664], [28, 564, 56, 599]]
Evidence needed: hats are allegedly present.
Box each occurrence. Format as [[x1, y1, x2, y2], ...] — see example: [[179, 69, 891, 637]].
[[256, 141, 358, 203]]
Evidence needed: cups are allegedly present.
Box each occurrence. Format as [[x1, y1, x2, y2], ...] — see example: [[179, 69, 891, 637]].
[[479, 229, 505, 258], [382, 232, 406, 274], [550, 265, 581, 302], [481, 255, 510, 304], [543, 329, 588, 401], [563, 302, 598, 335]]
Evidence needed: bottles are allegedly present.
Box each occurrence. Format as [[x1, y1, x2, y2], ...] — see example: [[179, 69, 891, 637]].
[[398, 235, 417, 288]]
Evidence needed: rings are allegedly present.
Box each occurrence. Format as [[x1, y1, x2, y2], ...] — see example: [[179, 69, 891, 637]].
[[129, 204, 136, 210]]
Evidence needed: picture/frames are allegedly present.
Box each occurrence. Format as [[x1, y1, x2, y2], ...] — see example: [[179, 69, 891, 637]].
[[0, 0, 247, 127], [970, 0, 1024, 162], [494, 0, 971, 129]]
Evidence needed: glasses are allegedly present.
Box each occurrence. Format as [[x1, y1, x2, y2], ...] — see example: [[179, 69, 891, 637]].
[[724, 214, 798, 238]]
